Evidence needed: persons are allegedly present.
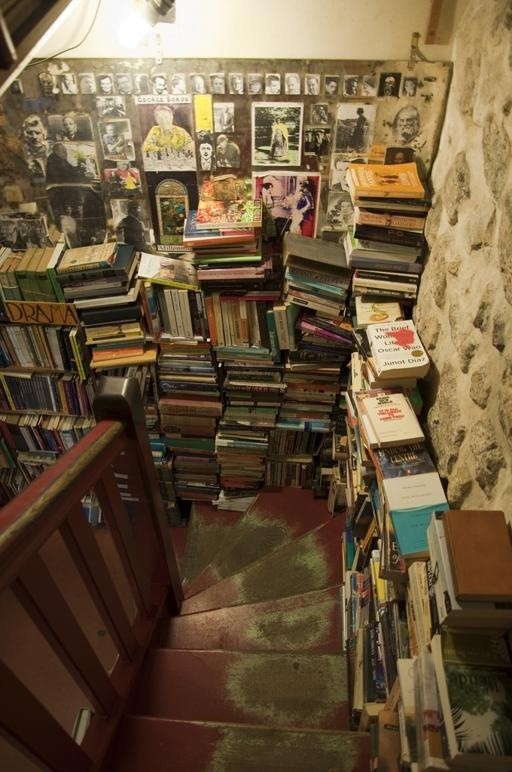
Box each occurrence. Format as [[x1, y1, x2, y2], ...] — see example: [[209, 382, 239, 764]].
[[20, 114, 49, 174], [102, 124, 128, 159], [215, 134, 240, 168], [270, 118, 289, 160], [218, 105, 234, 133], [102, 99, 125, 117], [62, 117, 91, 141], [308, 131, 329, 156], [392, 105, 420, 144], [350, 108, 369, 152], [142, 105, 196, 161], [196, 131, 216, 170], [314, 106, 328, 124]]
